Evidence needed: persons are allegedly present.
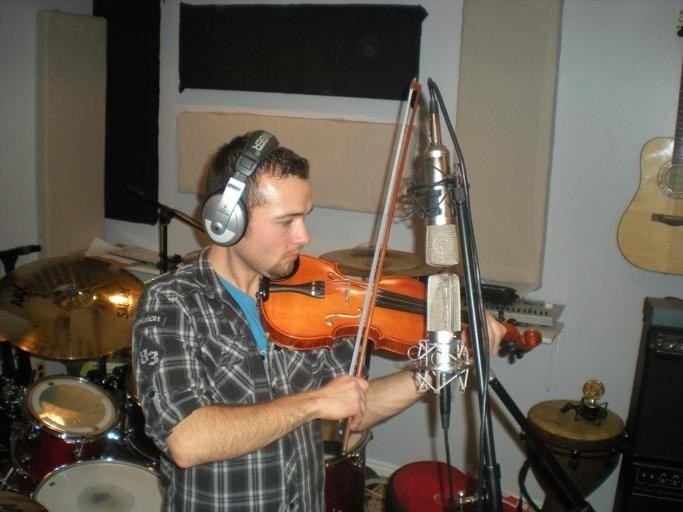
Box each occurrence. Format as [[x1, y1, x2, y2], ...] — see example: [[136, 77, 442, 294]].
[[132, 130, 508, 512]]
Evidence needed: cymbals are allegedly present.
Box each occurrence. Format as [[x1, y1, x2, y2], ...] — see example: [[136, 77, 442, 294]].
[[1, 256, 144, 360], [0, 490, 49, 512], [319, 249, 443, 276]]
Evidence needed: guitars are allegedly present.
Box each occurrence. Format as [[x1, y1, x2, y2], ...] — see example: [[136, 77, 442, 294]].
[[617, 8, 683, 273]]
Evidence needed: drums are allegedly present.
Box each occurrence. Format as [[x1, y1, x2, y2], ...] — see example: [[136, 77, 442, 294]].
[[122, 362, 164, 465], [1, 376, 120, 494], [525, 399, 625, 510], [322, 422, 374, 511], [38, 459, 167, 512]]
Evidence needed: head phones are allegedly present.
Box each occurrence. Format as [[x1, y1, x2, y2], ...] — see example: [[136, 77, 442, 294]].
[[201, 130, 280, 247]]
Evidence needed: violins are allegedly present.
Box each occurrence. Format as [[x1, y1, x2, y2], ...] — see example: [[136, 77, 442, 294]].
[[257, 253, 542, 363]]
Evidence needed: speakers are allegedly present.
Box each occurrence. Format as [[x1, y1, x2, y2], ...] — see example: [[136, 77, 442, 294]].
[[614, 325, 681, 511]]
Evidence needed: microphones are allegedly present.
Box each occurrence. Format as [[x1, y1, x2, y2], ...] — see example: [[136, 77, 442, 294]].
[[426, 274, 461, 430], [425, 101, 459, 270]]
